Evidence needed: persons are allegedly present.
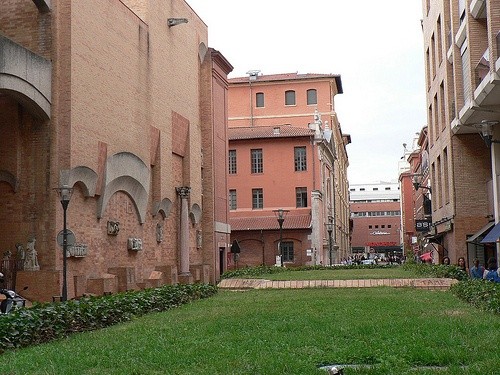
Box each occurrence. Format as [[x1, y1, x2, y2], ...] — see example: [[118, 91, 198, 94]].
[[441, 256, 450, 265], [343, 252, 367, 262], [380, 253, 403, 265], [469, 258, 485, 278], [458, 257, 470, 276], [483, 257, 500, 283]]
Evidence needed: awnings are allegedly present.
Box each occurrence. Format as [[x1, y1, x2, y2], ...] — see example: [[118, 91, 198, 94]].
[[465, 222, 496, 249], [481, 221, 500, 244], [426, 232, 448, 255]]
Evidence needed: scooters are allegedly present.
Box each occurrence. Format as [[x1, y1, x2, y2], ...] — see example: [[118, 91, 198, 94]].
[[0, 286, 29, 314]]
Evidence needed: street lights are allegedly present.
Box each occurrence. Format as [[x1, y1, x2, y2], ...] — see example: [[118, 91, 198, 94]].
[[272, 208, 290, 269], [324, 222, 337, 269], [56, 185, 75, 302]]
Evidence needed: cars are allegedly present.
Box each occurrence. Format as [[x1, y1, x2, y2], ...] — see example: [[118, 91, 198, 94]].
[[360, 260, 374, 265]]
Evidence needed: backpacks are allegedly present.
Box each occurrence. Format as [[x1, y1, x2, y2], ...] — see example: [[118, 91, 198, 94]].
[[486, 267, 500, 283]]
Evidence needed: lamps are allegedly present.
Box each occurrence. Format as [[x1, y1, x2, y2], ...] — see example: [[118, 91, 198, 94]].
[[409, 177, 432, 193], [475, 119, 500, 148]]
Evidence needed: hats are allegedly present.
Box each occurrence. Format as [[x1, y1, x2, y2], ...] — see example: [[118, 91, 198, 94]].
[[488, 257, 496, 264]]
